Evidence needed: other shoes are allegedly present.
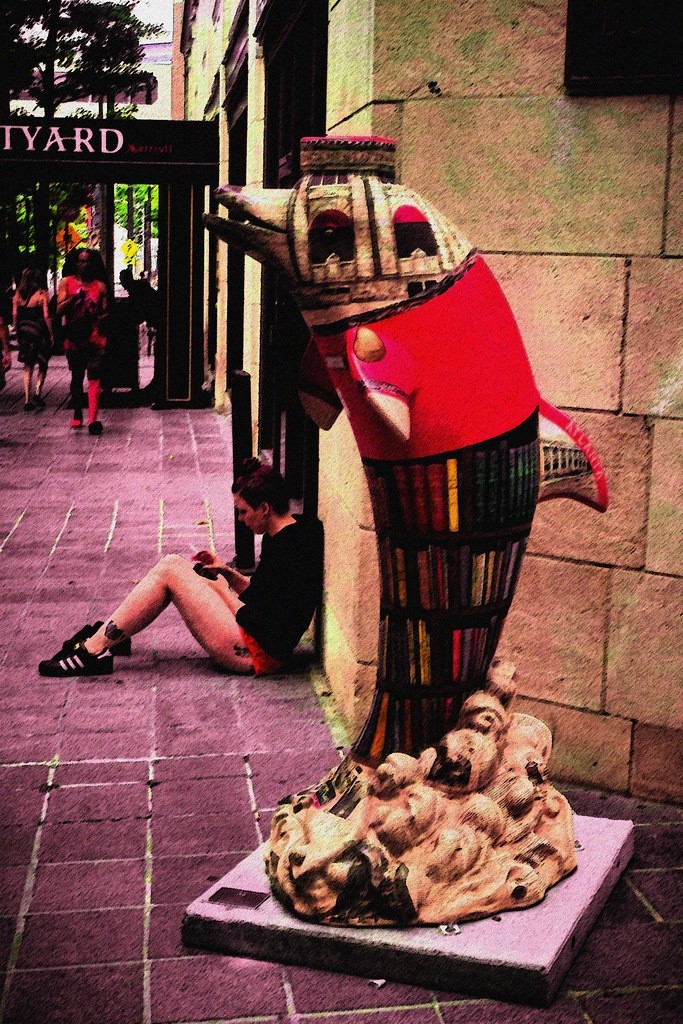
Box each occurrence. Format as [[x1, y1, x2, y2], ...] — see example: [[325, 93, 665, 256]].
[[70, 415, 82, 428], [31, 393, 45, 407], [24, 402, 36, 412], [88, 420, 103, 433]]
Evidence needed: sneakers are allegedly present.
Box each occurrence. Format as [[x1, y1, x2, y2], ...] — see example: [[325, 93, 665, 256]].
[[38, 639, 113, 677], [62, 620, 132, 656]]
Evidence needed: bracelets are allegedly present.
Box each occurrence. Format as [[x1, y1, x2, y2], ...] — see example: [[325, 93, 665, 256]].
[[228, 575, 246, 591]]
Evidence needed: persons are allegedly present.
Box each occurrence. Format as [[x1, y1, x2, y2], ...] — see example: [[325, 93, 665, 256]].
[[37, 457, 325, 678], [0, 250, 158, 434]]
